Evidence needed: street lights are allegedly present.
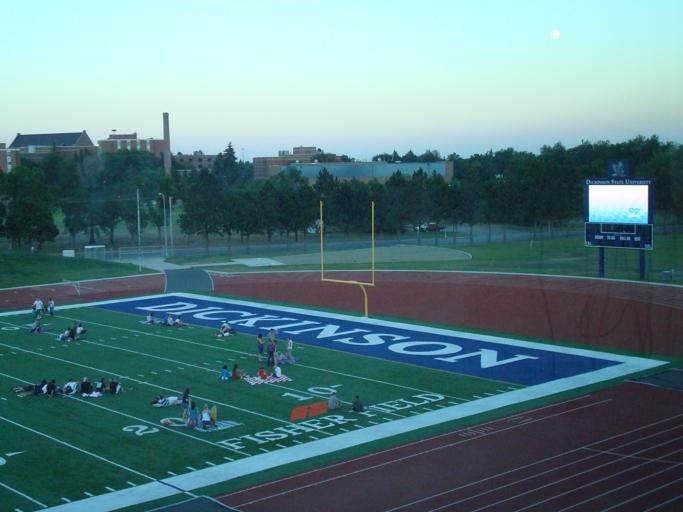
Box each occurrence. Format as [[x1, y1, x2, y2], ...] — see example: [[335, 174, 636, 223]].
[[157, 192, 169, 258]]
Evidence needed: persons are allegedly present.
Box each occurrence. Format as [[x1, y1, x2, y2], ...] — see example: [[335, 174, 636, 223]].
[[220, 364, 246, 380], [217, 323, 236, 338], [144, 312, 189, 326], [151, 388, 217, 430], [256, 329, 295, 380], [13, 377, 123, 398], [30, 297, 85, 343], [328, 392, 342, 409], [352, 395, 364, 412]]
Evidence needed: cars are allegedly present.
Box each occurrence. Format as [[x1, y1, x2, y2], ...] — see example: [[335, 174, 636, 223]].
[[415, 221, 447, 232]]
